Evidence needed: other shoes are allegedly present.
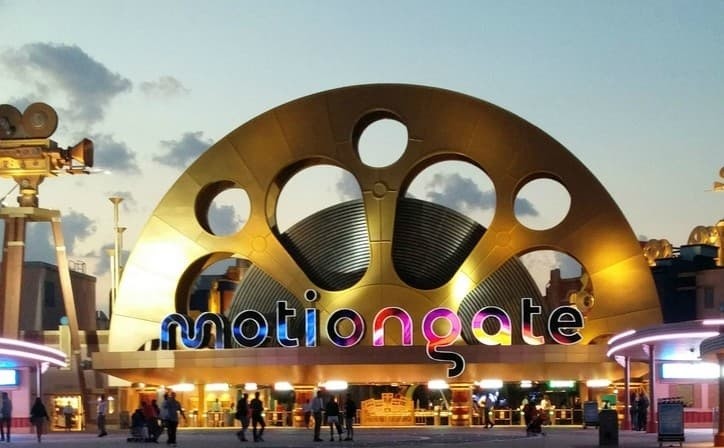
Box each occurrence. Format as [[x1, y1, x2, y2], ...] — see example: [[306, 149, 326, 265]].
[[241, 439, 248, 442], [253, 438, 259, 443], [7, 439, 10, 442], [153, 438, 159, 444], [236, 432, 240, 438], [258, 437, 264, 441], [313, 438, 323, 442], [99, 432, 107, 437], [339, 438, 342, 441], [484, 426, 488, 428], [0, 438, 4, 441], [166, 440, 176, 444], [329, 438, 334, 441], [343, 437, 352, 440], [490, 423, 494, 428]]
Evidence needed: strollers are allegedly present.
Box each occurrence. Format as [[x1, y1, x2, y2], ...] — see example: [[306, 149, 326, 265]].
[[526, 414, 548, 437]]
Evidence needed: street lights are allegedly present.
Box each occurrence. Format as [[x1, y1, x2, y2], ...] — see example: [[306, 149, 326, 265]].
[[105, 197, 126, 314]]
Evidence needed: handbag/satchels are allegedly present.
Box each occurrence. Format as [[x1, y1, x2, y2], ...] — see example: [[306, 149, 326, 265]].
[[235, 412, 240, 420], [30, 415, 34, 425]]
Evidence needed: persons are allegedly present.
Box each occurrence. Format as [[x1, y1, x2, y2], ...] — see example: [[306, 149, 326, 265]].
[[212, 398, 221, 427], [519, 394, 552, 438], [127, 392, 187, 445], [629, 390, 649, 431], [234, 391, 267, 444], [479, 393, 495, 431], [28, 395, 50, 445], [302, 388, 357, 441], [277, 400, 293, 426], [0, 390, 13, 445], [61, 398, 76, 434], [94, 393, 110, 439]]
